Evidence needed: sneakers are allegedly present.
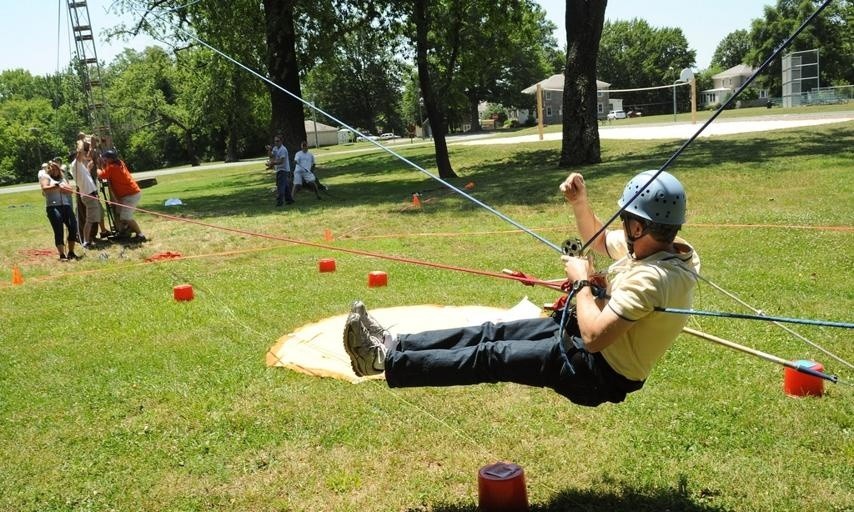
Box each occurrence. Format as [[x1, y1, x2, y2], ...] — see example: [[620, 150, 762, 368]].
[[276, 196, 321, 206], [59, 251, 82, 262], [343, 300, 390, 376], [83, 230, 145, 249]]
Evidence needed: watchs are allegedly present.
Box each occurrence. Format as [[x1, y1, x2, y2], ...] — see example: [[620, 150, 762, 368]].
[[573, 280, 591, 291]]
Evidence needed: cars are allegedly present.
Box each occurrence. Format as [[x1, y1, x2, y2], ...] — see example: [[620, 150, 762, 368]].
[[356, 133, 400, 142], [607, 110, 642, 121]]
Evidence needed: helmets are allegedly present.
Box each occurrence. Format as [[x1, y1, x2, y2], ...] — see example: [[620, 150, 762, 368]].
[[618, 169, 687, 224]]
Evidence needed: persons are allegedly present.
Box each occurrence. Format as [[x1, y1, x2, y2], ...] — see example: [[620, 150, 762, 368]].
[[39, 162, 82, 261], [51, 157, 67, 178], [97, 151, 146, 243], [68, 140, 116, 248], [291, 141, 322, 200], [343, 170, 701, 406], [265, 135, 295, 207], [38, 163, 48, 182]]
